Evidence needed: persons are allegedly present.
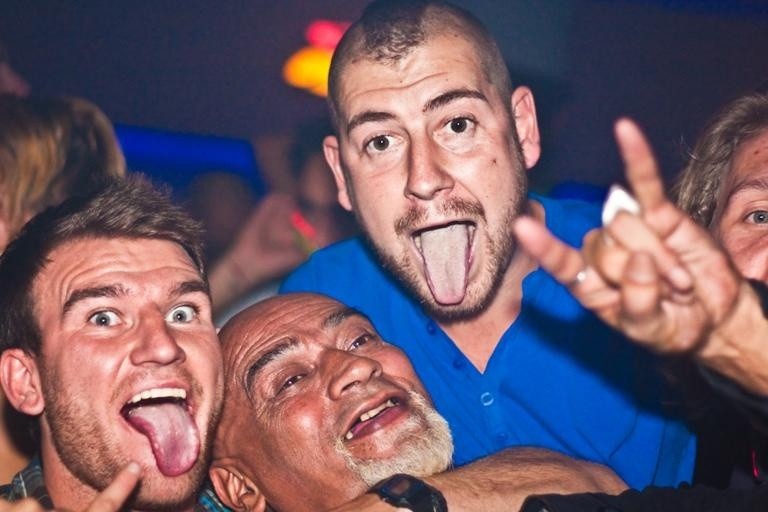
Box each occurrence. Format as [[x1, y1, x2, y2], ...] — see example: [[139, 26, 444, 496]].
[[191, 128, 356, 331], [2, 94, 126, 254], [273, 1, 767, 488], [673, 93, 767, 286], [208, 292, 629, 510], [0, 173, 629, 511]]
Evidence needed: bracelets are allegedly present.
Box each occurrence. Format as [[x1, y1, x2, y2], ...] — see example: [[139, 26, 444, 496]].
[[367, 472, 448, 511]]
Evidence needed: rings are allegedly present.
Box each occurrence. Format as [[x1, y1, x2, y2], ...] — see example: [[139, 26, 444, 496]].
[[563, 250, 591, 287]]
